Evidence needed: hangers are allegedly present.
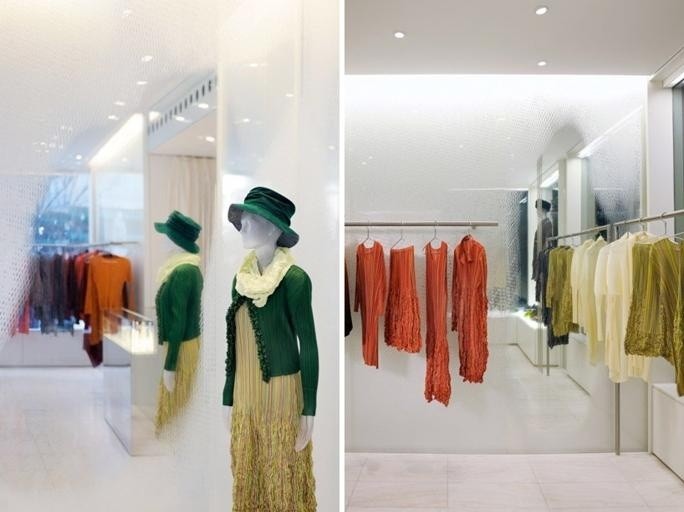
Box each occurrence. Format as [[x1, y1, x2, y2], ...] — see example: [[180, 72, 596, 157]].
[[357, 220, 470, 254], [551, 209, 684, 247]]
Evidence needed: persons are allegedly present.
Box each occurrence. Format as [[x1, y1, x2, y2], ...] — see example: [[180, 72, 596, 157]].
[[150, 209, 204, 441], [531, 199, 555, 321], [223, 186, 319, 512]]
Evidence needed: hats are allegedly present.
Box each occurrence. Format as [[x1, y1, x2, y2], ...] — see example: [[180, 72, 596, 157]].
[[535, 198, 551, 212], [153, 210, 202, 255], [227, 186, 300, 248]]
